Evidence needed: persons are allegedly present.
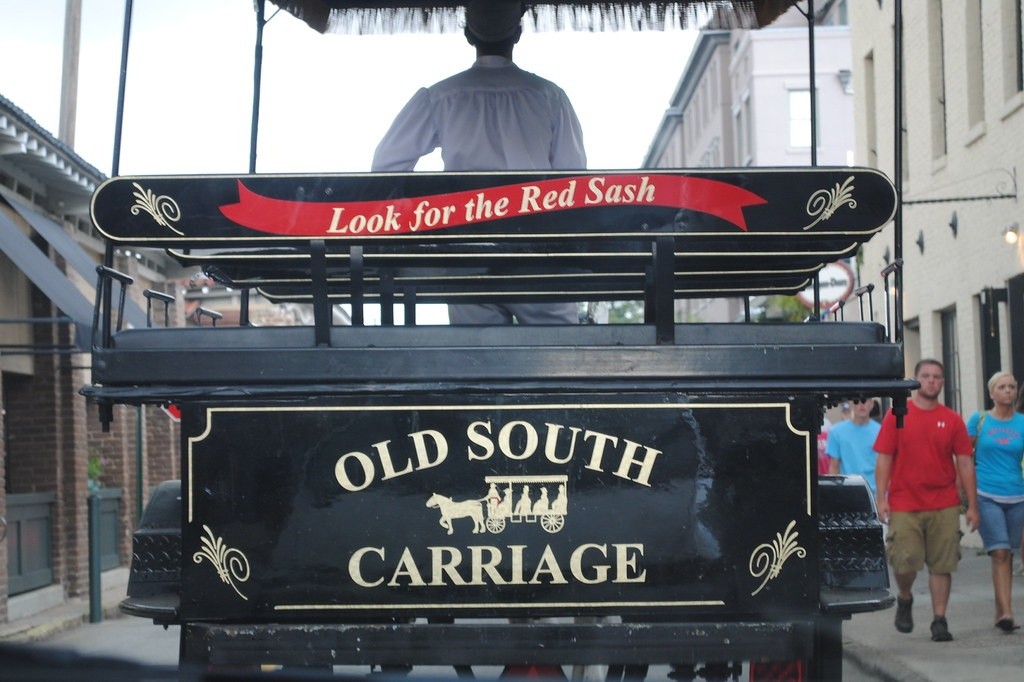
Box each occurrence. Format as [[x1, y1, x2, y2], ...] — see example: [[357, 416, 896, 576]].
[[817, 397, 852, 475], [869, 399, 883, 424], [966, 371, 1024, 631], [872, 360, 978, 642], [824, 396, 890, 551], [371, 2, 590, 328]]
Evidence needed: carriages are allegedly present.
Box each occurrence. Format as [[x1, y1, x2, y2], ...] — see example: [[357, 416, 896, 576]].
[[79, 0, 922, 682]]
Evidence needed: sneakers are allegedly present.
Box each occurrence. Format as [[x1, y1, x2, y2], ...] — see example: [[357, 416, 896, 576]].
[[895, 592, 913, 632], [930, 619, 952, 641]]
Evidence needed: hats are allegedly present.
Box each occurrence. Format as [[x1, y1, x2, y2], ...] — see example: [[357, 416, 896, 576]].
[[467, 0, 522, 42]]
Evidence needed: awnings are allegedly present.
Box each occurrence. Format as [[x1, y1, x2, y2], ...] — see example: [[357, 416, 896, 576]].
[[0, 208, 118, 338], [0, 190, 164, 330]]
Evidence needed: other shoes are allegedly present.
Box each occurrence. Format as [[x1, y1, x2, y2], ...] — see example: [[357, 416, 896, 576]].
[[993, 612, 1020, 630]]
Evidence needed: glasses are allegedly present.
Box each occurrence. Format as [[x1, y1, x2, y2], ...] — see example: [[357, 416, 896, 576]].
[[852, 398, 866, 404]]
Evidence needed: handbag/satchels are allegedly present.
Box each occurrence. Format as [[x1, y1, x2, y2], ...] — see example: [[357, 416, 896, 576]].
[[959, 513, 984, 550]]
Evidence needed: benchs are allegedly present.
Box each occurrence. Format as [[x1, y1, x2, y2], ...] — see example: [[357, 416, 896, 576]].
[[75, 160, 922, 437]]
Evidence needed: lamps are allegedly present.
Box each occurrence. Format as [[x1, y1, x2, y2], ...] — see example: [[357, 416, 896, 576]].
[[838, 68, 854, 95], [1004, 222, 1021, 243]]
